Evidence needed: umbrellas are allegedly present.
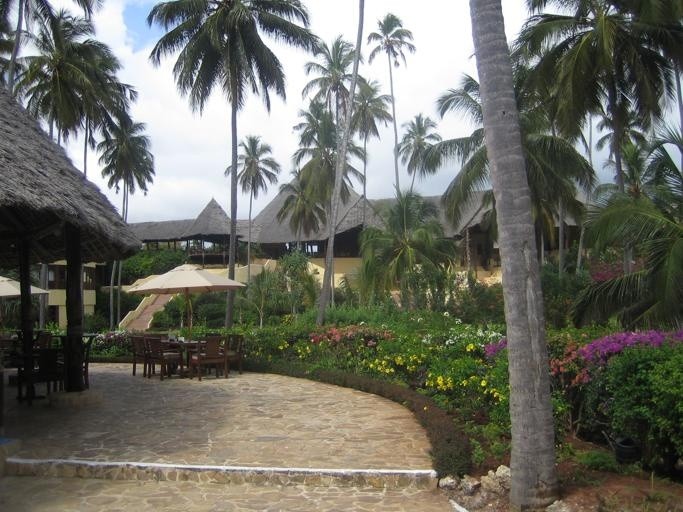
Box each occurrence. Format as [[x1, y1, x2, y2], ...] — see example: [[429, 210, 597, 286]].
[[127, 258, 247, 366]]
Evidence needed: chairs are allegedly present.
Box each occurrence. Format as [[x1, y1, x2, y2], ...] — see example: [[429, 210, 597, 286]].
[[0, 330, 243, 406]]
